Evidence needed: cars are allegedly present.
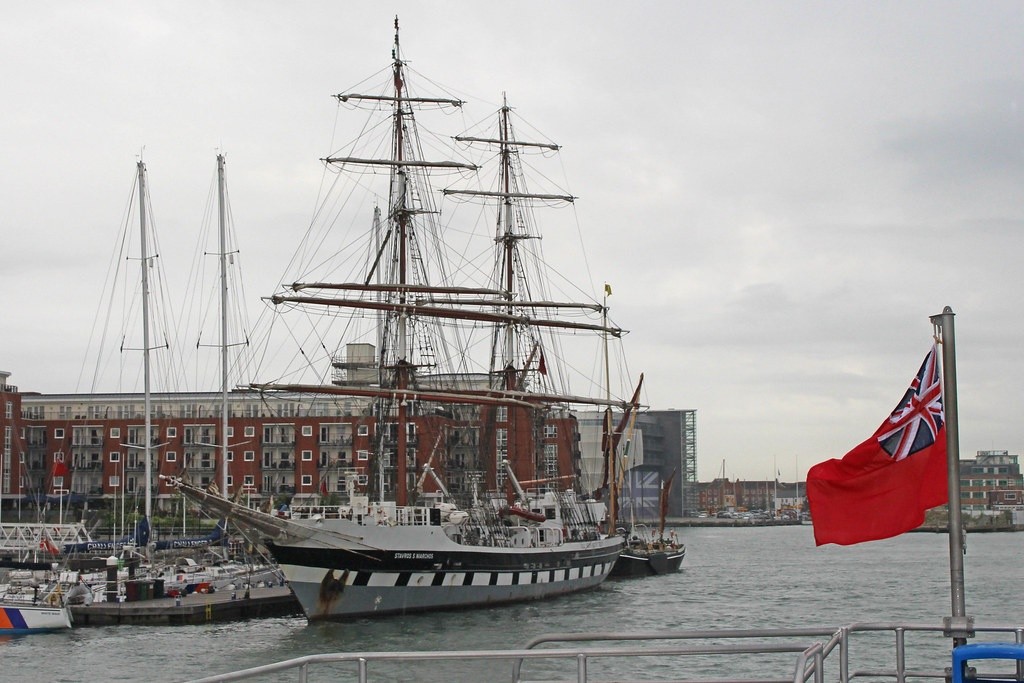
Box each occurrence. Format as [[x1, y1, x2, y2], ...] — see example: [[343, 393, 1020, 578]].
[[689, 509, 771, 520]]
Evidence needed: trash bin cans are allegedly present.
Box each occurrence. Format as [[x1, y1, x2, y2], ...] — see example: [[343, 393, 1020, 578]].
[[125, 577, 165, 602]]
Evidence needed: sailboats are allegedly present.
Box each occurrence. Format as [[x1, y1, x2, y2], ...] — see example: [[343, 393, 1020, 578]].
[[0, 15, 685, 635]]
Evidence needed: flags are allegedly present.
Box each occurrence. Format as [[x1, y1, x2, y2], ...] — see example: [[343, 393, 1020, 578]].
[[806, 344, 950, 547]]
